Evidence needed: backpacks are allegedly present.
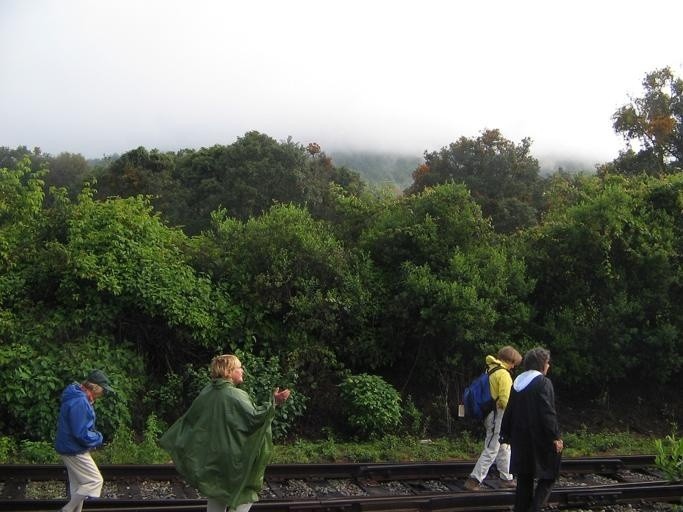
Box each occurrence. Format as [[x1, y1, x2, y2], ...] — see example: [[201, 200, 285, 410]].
[[463, 366, 511, 419]]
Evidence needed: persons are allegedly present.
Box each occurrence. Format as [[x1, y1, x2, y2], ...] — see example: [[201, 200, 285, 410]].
[[464, 346, 523, 492], [159, 354, 291, 512], [55, 371, 115, 512], [500, 346, 563, 512]]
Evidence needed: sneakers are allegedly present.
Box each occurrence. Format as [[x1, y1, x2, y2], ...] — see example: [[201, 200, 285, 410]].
[[464, 479, 480, 491], [497, 479, 517, 488]]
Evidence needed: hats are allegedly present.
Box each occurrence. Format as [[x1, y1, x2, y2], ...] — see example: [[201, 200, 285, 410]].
[[87, 370, 114, 392]]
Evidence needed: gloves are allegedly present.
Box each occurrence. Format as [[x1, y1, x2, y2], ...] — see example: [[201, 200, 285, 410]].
[[102, 434, 113, 442]]
